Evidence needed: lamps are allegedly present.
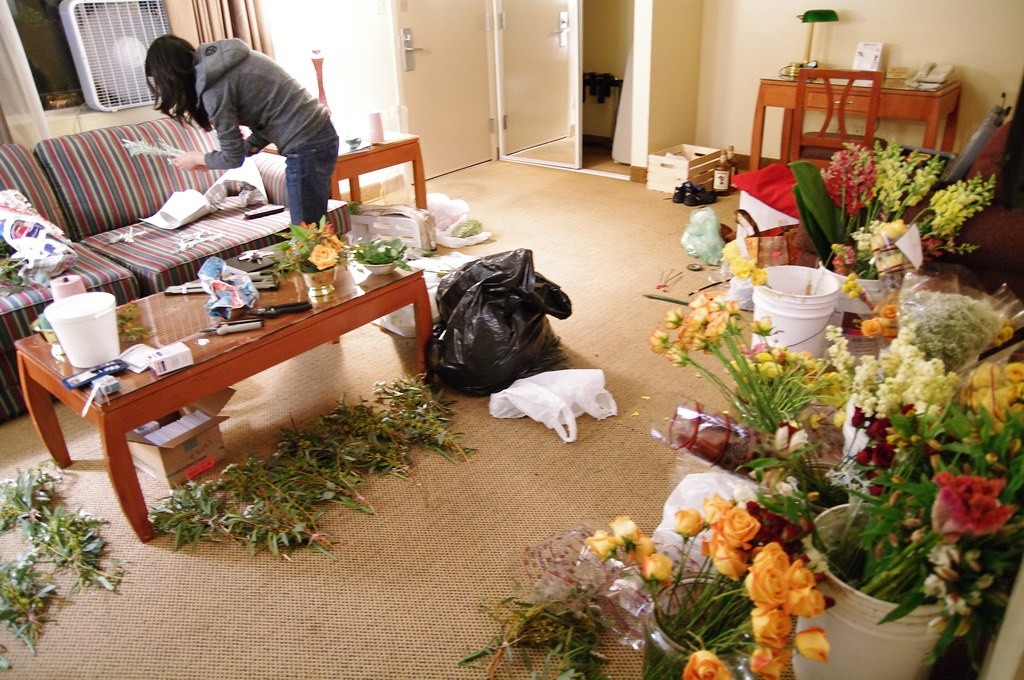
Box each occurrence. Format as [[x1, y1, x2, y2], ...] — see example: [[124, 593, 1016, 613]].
[[777, 7, 840, 81]]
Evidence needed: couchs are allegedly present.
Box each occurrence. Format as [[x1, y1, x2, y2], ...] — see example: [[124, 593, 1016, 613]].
[[0, 116, 351, 429]]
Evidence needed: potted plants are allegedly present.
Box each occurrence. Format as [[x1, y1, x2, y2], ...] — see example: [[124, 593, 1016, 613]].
[[353, 233, 413, 277]]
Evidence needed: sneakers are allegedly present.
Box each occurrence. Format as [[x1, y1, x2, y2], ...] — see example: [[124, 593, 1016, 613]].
[[685, 188, 717, 206], [673, 181, 701, 204]]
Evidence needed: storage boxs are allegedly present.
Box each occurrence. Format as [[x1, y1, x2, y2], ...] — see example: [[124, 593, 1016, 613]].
[[734, 188, 801, 256], [125, 387, 239, 490], [644, 140, 722, 196], [145, 340, 197, 377], [851, 40, 884, 85]]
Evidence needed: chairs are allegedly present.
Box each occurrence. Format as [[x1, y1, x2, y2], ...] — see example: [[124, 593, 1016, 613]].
[[789, 64, 889, 170]]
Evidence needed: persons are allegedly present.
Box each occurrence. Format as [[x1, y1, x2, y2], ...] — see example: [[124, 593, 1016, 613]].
[[145, 34, 339, 231]]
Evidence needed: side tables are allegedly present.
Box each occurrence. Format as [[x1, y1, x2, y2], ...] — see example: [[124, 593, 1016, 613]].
[[264, 112, 430, 214]]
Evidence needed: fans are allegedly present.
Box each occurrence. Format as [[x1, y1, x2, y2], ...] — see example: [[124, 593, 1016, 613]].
[[56, 0, 176, 113]]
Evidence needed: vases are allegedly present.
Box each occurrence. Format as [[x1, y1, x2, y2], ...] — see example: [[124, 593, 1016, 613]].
[[299, 268, 339, 299], [641, 570, 775, 680]]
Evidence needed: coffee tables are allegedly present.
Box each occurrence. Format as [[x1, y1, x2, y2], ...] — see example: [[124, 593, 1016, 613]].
[[13, 242, 438, 542]]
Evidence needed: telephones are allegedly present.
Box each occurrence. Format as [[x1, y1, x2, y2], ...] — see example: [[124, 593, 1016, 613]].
[[916, 62, 954, 82]]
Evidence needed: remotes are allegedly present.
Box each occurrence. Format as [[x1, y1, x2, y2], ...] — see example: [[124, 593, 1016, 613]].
[[244, 205, 285, 219]]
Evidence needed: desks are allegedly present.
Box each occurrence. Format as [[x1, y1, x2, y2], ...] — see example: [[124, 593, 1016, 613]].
[[747, 73, 965, 172]]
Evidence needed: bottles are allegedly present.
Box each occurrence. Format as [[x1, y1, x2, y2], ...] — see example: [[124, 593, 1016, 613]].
[[726, 145, 739, 192], [712, 149, 731, 196], [368, 112, 385, 143]]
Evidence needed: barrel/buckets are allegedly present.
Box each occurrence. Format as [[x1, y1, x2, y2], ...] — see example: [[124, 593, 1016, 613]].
[[42, 291, 120, 369], [818, 260, 887, 360], [751, 265, 840, 357], [791, 503, 949, 680]]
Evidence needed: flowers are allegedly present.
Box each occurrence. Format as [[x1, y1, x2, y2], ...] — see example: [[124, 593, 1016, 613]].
[[270, 215, 352, 276], [582, 133, 1024, 680]]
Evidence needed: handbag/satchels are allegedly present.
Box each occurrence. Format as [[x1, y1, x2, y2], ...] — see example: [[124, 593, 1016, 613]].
[[489, 369, 617, 445], [726, 209, 789, 269]]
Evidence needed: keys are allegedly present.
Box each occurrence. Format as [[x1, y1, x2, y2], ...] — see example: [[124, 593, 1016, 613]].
[[238, 250, 274, 265]]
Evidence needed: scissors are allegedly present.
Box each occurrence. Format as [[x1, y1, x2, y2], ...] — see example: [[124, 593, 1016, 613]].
[[247, 300, 314, 318], [199, 317, 263, 336]]
